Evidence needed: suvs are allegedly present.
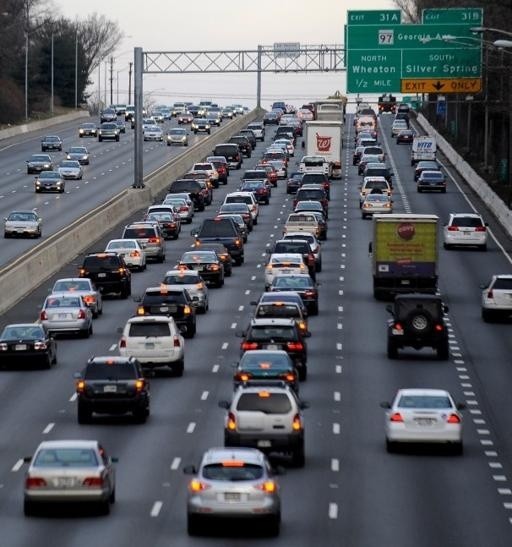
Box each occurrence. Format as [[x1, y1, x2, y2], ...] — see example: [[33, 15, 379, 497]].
[[191, 218, 249, 265], [204, 107, 223, 122], [248, 122, 265, 142], [275, 233, 321, 270], [255, 164, 278, 187], [293, 188, 328, 218], [387, 294, 449, 358], [78, 253, 131, 298], [264, 253, 309, 290], [121, 221, 168, 263], [393, 120, 407, 137], [125, 105, 146, 121], [73, 357, 155, 424], [234, 105, 244, 115], [212, 144, 246, 169], [297, 109, 313, 121], [395, 113, 409, 127], [97, 122, 120, 142], [167, 181, 206, 211], [143, 205, 180, 233], [270, 102, 287, 113], [116, 316, 187, 377], [188, 163, 219, 188], [218, 380, 310, 465], [239, 130, 257, 150], [238, 318, 312, 365], [358, 146, 388, 176], [133, 287, 199, 339], [228, 136, 252, 158], [187, 106, 200, 117]]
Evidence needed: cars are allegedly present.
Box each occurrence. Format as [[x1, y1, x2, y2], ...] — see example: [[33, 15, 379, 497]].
[[239, 180, 271, 204], [253, 303, 305, 334], [262, 148, 286, 160], [264, 161, 288, 179], [263, 112, 279, 125], [35, 171, 66, 193], [143, 126, 163, 141], [183, 446, 282, 536], [234, 349, 298, 394], [265, 275, 319, 313], [211, 162, 230, 184], [256, 134, 293, 187], [206, 115, 221, 126], [184, 173, 212, 204], [355, 139, 378, 147], [414, 160, 443, 183], [191, 119, 210, 134], [271, 108, 284, 115], [23, 441, 118, 514], [161, 267, 210, 313], [56, 160, 82, 179], [177, 111, 194, 124], [176, 251, 223, 288], [101, 239, 146, 271], [142, 118, 157, 132], [354, 103, 379, 138], [379, 388, 466, 454], [286, 172, 303, 192], [267, 241, 318, 280], [166, 128, 188, 146], [397, 103, 409, 113], [220, 192, 258, 225], [39, 295, 95, 338], [221, 108, 233, 119], [279, 115, 303, 136], [115, 105, 127, 114], [161, 193, 194, 216], [398, 130, 413, 144], [363, 163, 390, 177], [416, 170, 447, 194], [66, 147, 90, 165], [144, 212, 178, 240], [149, 112, 167, 123], [0, 324, 56, 368], [41, 135, 63, 151], [216, 204, 252, 232], [361, 195, 393, 217], [249, 293, 306, 316], [113, 121, 126, 133], [206, 156, 229, 175], [190, 244, 232, 276], [160, 199, 192, 224], [444, 213, 487, 251], [352, 147, 366, 165], [3, 208, 42, 238], [52, 279, 104, 318], [213, 214, 247, 243], [26, 154, 54, 174], [479, 274, 512, 321], [78, 123, 97, 137], [293, 201, 327, 221]]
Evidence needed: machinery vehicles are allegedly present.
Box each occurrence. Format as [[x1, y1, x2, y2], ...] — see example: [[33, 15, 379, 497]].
[[378, 93, 396, 115]]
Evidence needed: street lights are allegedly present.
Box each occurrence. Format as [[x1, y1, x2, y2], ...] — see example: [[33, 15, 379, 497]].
[[442, 33, 489, 174], [469, 26, 512, 35]]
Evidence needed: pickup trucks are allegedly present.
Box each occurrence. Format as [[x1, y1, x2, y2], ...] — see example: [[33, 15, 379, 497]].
[[240, 170, 273, 197], [285, 213, 320, 239], [295, 155, 332, 176]]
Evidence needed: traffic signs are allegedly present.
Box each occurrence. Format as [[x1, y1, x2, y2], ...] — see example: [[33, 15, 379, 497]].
[[344, 9, 483, 94]]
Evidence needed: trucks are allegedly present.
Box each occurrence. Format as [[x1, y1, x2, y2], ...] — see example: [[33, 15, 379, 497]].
[[302, 121, 341, 179], [174, 102, 187, 117], [314, 100, 345, 127], [410, 137, 438, 166], [369, 215, 440, 299]]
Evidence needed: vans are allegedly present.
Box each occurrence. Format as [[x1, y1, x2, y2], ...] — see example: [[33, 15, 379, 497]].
[[100, 109, 117, 123], [199, 182, 209, 198], [301, 172, 333, 200], [359, 177, 393, 207], [159, 108, 171, 119], [226, 106, 236, 115], [274, 126, 297, 148]]
